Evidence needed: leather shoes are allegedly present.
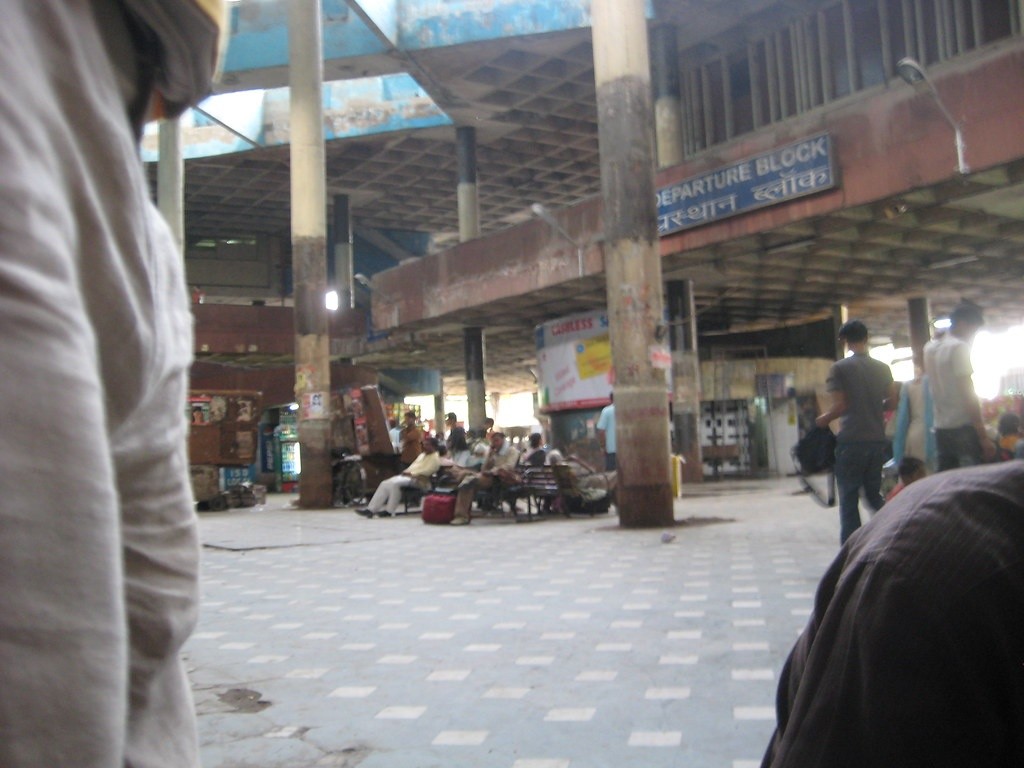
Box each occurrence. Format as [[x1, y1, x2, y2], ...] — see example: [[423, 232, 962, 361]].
[[355, 509, 374, 519], [375, 510, 393, 517]]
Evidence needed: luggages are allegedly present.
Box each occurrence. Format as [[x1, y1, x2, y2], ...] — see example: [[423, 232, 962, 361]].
[[422, 493, 456, 523]]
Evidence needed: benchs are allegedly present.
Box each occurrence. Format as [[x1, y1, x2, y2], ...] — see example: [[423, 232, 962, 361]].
[[429, 466, 541, 523], [518, 465, 594, 518], [358, 469, 438, 514]]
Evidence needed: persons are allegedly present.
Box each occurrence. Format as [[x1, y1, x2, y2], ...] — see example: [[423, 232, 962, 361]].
[[760, 459, 1024, 768], [920, 305, 997, 472], [342, 411, 617, 525], [880, 351, 1024, 499], [813, 317, 896, 547], [191, 405, 205, 423], [0, 0, 230, 768], [596, 392, 618, 504]]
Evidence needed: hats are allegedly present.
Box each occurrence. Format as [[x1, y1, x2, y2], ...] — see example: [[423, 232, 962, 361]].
[[530, 434, 542, 449], [445, 412, 458, 421]]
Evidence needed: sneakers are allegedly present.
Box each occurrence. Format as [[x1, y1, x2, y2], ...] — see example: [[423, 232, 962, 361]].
[[450, 516, 471, 525]]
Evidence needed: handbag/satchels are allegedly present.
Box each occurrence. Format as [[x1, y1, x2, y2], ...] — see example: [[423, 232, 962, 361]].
[[790, 423, 836, 477], [498, 468, 529, 485]]
